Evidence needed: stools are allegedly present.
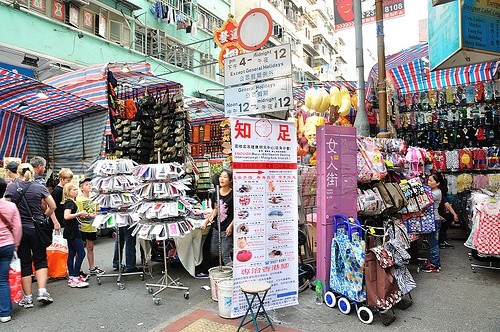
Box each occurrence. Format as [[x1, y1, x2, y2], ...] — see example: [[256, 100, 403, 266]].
[[236, 281, 275, 332]]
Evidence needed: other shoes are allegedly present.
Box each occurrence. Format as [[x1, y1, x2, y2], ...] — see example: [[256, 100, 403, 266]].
[[113, 263, 125, 268], [126, 266, 144, 273], [0, 315, 11, 322]]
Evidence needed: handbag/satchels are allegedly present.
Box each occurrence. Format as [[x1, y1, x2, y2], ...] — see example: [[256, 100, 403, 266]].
[[46, 228, 68, 279], [108, 71, 185, 162], [402, 190, 435, 221], [357, 185, 387, 216], [356, 137, 388, 183], [376, 183, 408, 210], [407, 201, 435, 234], [34, 218, 52, 245], [8, 250, 23, 304], [398, 176, 434, 214]]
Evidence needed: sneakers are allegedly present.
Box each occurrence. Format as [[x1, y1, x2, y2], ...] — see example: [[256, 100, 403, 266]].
[[90, 267, 105, 275], [439, 241, 454, 249], [69, 277, 89, 288], [19, 295, 34, 308], [422, 261, 441, 272], [195, 272, 209, 278], [68, 274, 87, 282], [38, 291, 54, 303]]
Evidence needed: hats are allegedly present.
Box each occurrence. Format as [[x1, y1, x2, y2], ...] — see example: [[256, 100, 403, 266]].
[[5, 161, 19, 169], [79, 176, 92, 187]]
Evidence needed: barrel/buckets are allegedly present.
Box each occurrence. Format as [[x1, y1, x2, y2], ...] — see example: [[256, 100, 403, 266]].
[[217, 278, 233, 319], [208, 266, 233, 301]]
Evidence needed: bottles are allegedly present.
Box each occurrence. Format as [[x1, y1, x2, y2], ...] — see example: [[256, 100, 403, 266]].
[[315, 280, 323, 305]]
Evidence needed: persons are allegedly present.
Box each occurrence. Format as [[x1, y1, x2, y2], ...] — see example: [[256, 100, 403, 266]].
[[112, 226, 144, 272], [192, 170, 236, 279], [420, 173, 459, 274], [0, 157, 105, 323]]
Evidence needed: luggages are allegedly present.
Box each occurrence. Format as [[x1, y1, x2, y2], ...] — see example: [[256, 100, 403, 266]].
[[324, 215, 417, 326]]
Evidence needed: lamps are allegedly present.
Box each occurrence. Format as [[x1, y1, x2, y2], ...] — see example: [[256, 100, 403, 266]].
[[7, 0, 20, 11], [49, 62, 76, 73], [21, 53, 39, 69], [36, 90, 49, 99], [18, 100, 28, 109], [78, 32, 84, 39]]
